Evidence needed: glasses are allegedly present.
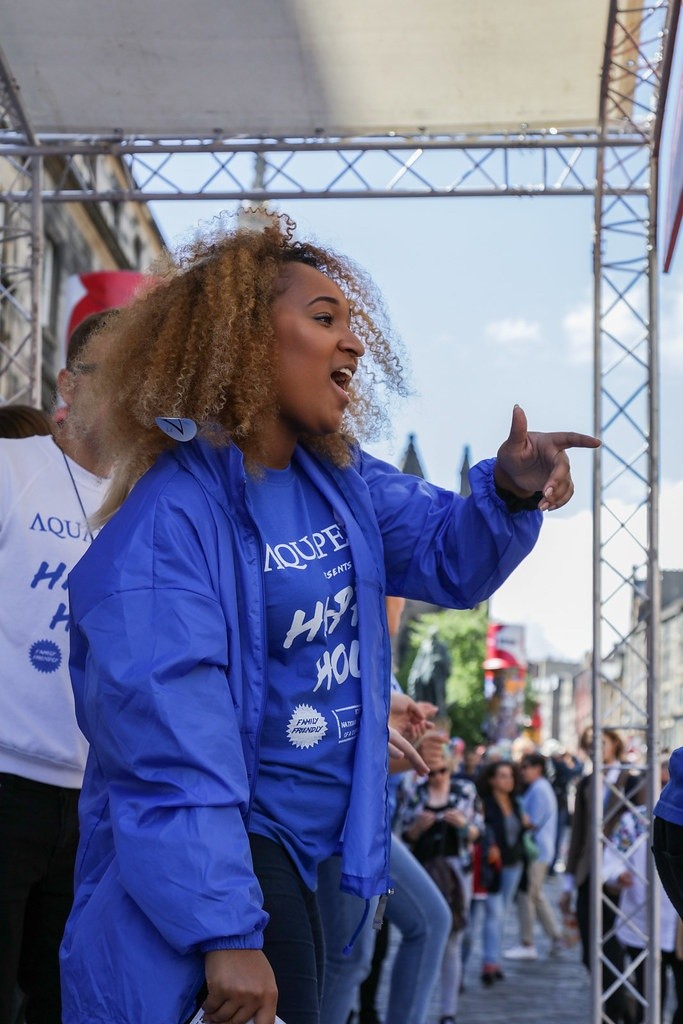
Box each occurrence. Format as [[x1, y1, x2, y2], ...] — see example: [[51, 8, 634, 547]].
[[428, 768, 449, 777]]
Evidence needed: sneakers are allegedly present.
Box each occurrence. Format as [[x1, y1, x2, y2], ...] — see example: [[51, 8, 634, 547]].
[[502, 945, 537, 961]]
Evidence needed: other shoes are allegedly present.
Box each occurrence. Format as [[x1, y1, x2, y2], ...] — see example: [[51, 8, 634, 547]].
[[484, 971, 504, 984], [550, 941, 571, 957]]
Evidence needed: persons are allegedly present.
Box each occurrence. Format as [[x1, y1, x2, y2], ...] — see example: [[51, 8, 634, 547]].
[[355, 594, 683, 1024], [0, 309, 115, 1024], [68, 207, 601, 1024]]
